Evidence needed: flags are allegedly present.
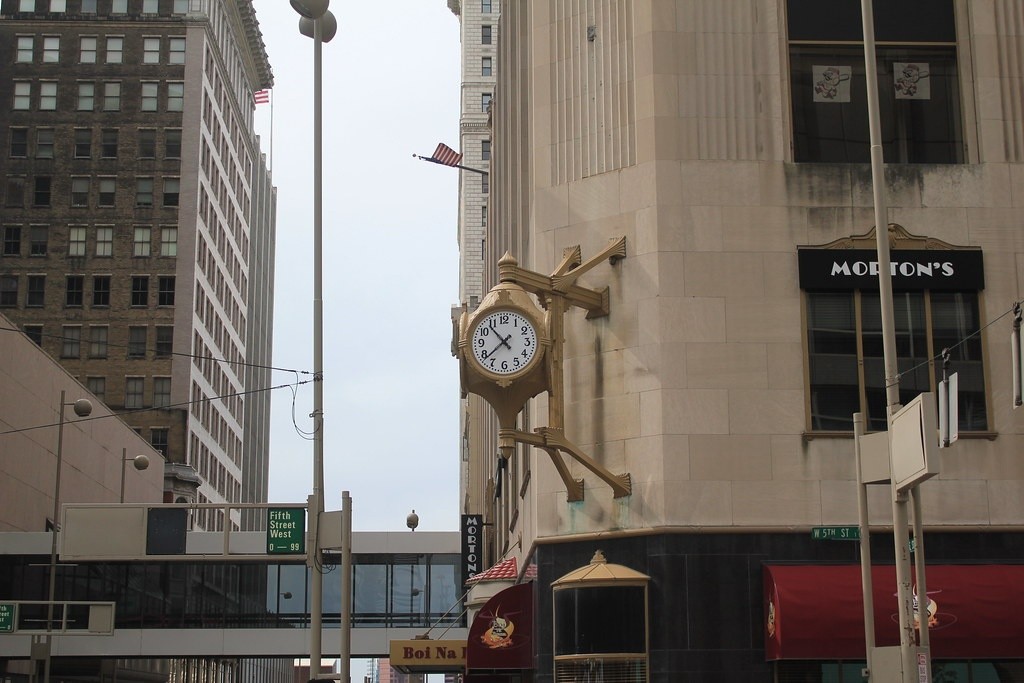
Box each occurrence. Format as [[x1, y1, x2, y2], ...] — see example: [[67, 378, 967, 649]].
[[418, 142, 464, 167], [254, 91, 269, 103]]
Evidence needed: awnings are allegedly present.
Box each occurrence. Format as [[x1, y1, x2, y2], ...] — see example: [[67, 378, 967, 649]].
[[464, 582, 535, 675], [761, 563, 1024, 661]]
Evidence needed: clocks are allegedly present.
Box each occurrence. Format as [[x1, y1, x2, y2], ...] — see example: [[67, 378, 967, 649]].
[[473, 308, 538, 376]]
